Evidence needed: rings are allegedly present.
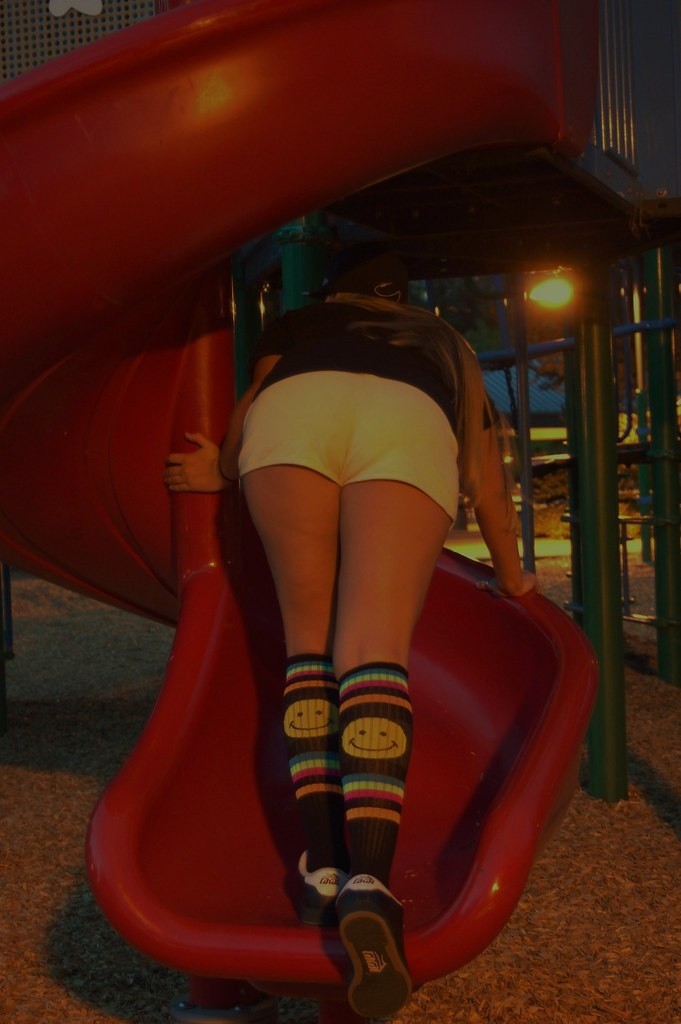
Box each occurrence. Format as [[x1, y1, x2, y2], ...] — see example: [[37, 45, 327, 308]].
[[485, 580, 489, 590]]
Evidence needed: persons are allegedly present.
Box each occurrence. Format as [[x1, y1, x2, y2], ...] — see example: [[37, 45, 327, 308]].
[[164, 293, 542, 1017]]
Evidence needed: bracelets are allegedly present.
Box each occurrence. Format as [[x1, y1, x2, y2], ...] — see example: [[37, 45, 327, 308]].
[[218, 438, 238, 482]]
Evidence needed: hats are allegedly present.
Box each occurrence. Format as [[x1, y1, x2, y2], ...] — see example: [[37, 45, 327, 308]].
[[300, 244, 408, 303]]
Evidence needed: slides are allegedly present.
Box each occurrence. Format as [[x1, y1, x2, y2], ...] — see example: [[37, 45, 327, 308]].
[[0, 0, 605, 990]]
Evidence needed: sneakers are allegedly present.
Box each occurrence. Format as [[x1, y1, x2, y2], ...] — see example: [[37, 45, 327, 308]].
[[334, 873, 412, 1019], [297, 847, 352, 926]]
